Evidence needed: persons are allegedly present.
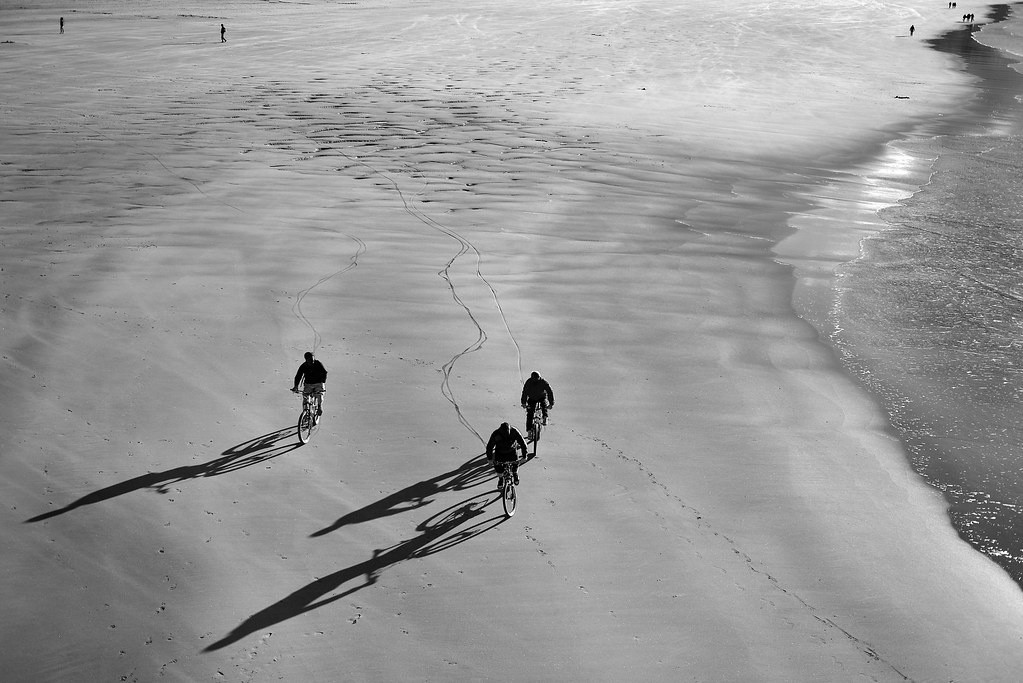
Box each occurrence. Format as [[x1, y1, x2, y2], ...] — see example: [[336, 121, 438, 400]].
[[59, 17, 64, 34], [486, 422, 528, 490], [962, 14, 974, 22], [521, 372, 554, 437], [949, 2, 956, 9], [909, 25, 915, 36], [220, 24, 227, 42], [292, 352, 327, 423]]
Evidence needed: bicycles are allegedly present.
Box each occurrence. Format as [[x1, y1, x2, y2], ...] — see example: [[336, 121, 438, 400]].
[[290, 387, 326, 444], [490, 456, 527, 517], [524, 400, 552, 456]]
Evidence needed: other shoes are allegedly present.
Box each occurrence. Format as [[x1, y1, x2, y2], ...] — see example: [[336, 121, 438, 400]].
[[513, 475, 519, 485], [528, 431, 531, 439], [497, 480, 503, 489], [542, 417, 548, 425], [318, 409, 322, 416]]
